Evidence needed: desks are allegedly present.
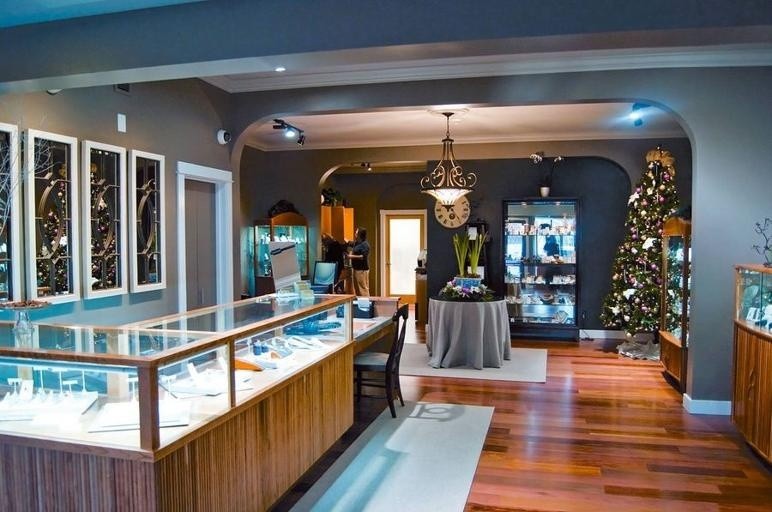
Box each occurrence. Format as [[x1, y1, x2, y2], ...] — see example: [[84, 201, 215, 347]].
[[328, 314, 393, 357], [426, 297, 511, 370]]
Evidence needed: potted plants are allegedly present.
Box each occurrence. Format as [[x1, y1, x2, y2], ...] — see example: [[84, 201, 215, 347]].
[[453, 232, 488, 287]]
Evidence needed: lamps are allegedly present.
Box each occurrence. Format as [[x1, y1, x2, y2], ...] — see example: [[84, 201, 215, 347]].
[[273, 119, 305, 146], [420, 113, 477, 212]]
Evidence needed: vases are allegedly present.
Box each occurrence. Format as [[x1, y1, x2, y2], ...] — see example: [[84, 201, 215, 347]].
[[541, 187, 550, 198]]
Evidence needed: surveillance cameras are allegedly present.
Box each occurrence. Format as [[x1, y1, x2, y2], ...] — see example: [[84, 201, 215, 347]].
[[217, 129, 231, 145]]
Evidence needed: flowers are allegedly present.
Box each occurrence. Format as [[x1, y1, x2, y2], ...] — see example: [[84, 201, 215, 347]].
[[528, 153, 565, 187], [441, 280, 495, 301]]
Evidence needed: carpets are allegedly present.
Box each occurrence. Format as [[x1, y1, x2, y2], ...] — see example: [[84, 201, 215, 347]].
[[289, 399, 495, 512], [399, 343, 548, 383]]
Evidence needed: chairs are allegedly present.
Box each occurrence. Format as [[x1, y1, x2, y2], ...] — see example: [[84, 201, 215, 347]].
[[354, 303, 408, 418]]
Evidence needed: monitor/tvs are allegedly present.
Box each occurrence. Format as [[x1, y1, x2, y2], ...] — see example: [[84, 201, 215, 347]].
[[313, 260, 338, 286]]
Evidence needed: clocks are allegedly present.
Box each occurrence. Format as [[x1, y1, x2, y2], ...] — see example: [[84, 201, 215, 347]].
[[434, 196, 471, 229]]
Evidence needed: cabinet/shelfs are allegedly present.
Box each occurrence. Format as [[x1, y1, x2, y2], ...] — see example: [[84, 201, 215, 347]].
[[254, 199, 309, 296], [658, 213, 691, 391], [502, 197, 582, 342], [0, 293, 356, 512], [730, 263, 772, 470], [322, 205, 354, 244]]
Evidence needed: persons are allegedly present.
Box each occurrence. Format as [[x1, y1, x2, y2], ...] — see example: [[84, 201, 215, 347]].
[[542, 223, 559, 255], [344, 226, 371, 295]]
